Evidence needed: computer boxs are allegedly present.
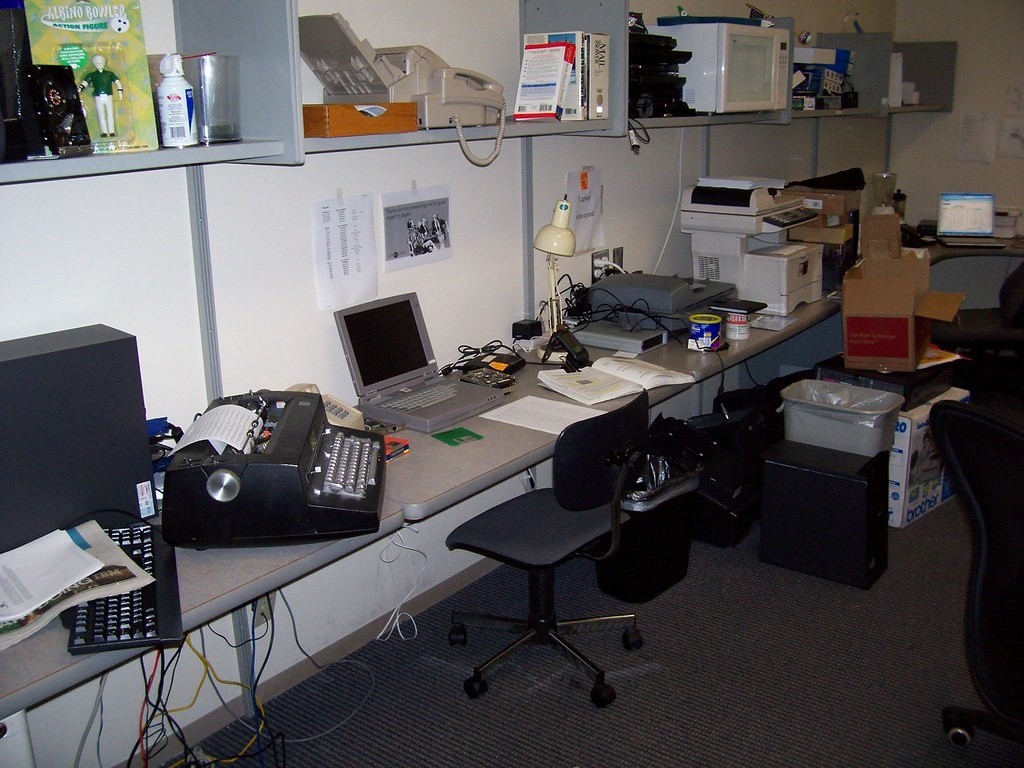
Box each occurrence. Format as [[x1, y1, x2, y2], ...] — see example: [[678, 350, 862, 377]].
[[759, 440, 888, 592], [0, 324, 160, 555]]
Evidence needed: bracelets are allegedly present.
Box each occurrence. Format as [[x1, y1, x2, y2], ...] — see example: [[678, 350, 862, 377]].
[[79, 84, 85, 91], [117, 88, 123, 92]]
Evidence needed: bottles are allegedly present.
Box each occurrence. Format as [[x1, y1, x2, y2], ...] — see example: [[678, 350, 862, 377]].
[[157, 53, 198, 146], [892, 189, 906, 219]]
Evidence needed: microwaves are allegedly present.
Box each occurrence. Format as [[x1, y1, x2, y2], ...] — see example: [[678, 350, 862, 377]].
[[642, 23, 789, 116]]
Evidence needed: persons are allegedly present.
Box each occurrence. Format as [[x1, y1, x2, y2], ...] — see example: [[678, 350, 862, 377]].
[[78, 55, 123, 138]]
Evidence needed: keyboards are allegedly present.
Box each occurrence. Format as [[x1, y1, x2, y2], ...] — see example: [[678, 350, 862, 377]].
[[68, 525, 183, 656], [322, 431, 381, 500]]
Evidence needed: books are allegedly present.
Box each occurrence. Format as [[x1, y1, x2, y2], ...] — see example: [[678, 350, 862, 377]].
[[696, 174, 786, 190], [0, 520, 156, 649], [916, 347, 962, 370], [514, 31, 609, 123], [537, 357, 694, 406]]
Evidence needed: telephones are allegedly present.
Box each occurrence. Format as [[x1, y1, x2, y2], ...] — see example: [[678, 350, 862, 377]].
[[428, 67, 505, 109]]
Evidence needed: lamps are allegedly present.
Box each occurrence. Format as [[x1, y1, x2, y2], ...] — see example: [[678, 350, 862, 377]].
[[533, 191, 575, 362]]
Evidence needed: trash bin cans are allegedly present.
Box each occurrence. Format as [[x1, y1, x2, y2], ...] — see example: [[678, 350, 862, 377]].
[[780, 379, 905, 454], [592, 473, 701, 605]]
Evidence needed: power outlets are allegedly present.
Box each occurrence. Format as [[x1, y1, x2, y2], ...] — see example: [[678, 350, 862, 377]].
[[591, 249, 610, 285]]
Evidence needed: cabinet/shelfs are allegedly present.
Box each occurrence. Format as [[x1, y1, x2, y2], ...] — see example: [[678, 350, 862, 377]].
[[0, 0, 1024, 768]]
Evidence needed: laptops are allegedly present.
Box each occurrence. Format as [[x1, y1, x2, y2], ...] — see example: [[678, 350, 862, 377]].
[[334, 292, 505, 433], [937, 191, 1006, 248]]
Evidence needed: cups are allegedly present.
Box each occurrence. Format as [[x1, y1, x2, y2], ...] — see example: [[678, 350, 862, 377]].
[[198, 57, 240, 141]]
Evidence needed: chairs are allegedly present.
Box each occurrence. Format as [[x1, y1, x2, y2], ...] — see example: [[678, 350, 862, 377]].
[[928, 399, 1024, 750], [444, 389, 649, 710]]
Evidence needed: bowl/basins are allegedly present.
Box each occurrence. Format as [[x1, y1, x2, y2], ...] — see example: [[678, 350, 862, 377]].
[[686, 313, 722, 351], [727, 322, 750, 340]]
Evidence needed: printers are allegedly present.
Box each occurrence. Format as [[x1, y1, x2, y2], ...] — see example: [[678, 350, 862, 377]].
[[680, 176, 825, 317]]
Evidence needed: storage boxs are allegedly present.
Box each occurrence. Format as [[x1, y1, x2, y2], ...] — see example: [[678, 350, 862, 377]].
[[302, 102, 419, 139], [800, 214, 848, 229], [842, 249, 967, 372], [781, 185, 859, 215], [888, 387, 969, 529], [793, 47, 857, 109], [861, 213, 902, 259], [779, 378, 906, 456], [789, 223, 853, 244]]
